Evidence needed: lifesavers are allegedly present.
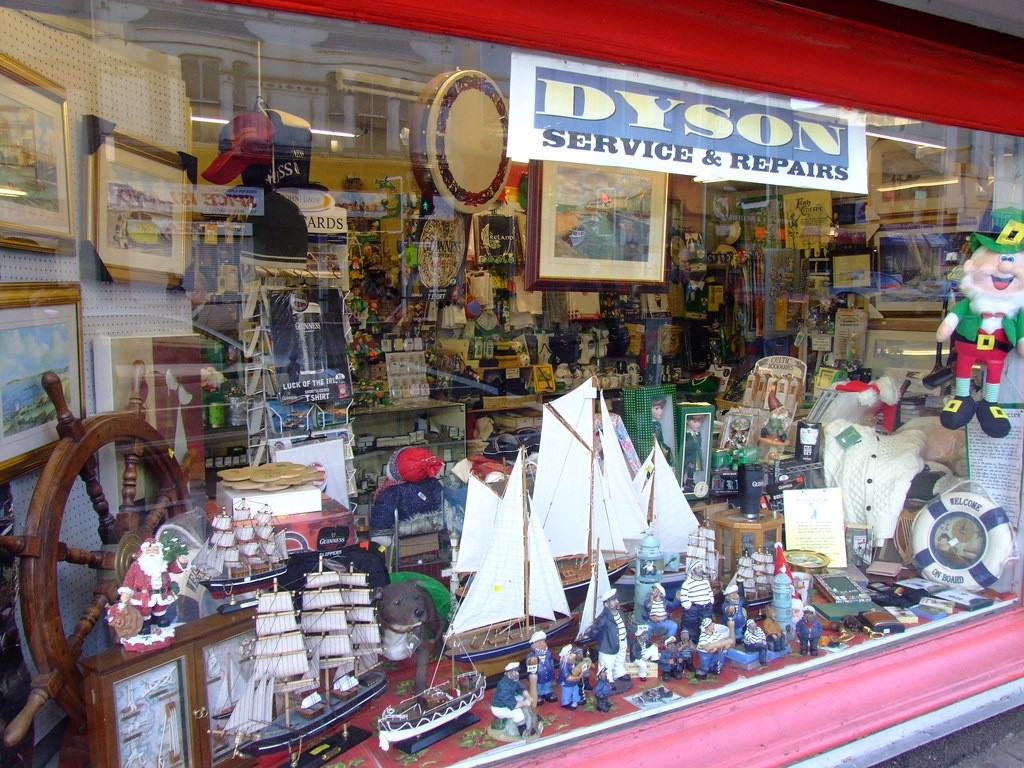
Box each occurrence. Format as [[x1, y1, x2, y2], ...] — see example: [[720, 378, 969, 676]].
[[908, 492, 1015, 590]]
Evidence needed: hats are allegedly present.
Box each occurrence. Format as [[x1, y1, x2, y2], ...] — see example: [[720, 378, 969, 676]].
[[830, 380, 880, 406], [201, 112, 276, 185], [387, 445, 444, 484]]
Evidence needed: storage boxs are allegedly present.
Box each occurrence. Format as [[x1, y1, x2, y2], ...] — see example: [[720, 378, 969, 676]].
[[215, 481, 323, 520], [621, 385, 637, 453], [635, 384, 678, 478], [207, 494, 354, 558], [709, 471, 739, 496], [678, 402, 716, 499]]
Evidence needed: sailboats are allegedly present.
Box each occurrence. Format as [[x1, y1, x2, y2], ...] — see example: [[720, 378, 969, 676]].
[[207, 550, 388, 759], [686, 524, 723, 606], [197, 497, 288, 607], [725, 544, 775, 608], [442, 367, 703, 684]]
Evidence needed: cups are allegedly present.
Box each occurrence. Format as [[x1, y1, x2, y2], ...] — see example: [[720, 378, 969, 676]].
[[226, 396, 247, 426], [610, 374, 631, 387], [824, 352, 839, 369], [631, 374, 643, 386]]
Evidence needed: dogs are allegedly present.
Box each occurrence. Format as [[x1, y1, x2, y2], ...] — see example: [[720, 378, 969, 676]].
[[371, 578, 451, 696]]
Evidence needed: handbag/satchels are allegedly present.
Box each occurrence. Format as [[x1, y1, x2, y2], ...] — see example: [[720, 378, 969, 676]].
[[466, 299, 482, 318], [241, 97, 312, 188], [476, 309, 497, 330]]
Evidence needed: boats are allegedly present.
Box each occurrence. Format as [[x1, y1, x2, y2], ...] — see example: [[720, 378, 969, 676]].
[[569, 226, 586, 246], [377, 624, 486, 752], [588, 215, 601, 226]]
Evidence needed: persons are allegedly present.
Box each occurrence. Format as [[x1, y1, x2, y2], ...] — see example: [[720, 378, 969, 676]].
[[685, 414, 704, 493], [118, 536, 189, 640], [935, 206, 1024, 439], [762, 390, 789, 442], [594, 430, 605, 475], [652, 396, 674, 469], [113, 214, 127, 251], [490, 560, 824, 738]]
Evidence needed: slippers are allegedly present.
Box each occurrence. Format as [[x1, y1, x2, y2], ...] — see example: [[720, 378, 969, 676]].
[[483, 434, 531, 459], [513, 427, 541, 452]]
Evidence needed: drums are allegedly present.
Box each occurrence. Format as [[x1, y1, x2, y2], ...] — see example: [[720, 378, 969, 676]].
[[408, 68, 511, 215]]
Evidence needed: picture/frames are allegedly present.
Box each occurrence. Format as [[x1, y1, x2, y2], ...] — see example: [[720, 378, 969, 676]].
[[827, 246, 879, 296], [79, 114, 198, 291], [738, 192, 786, 250], [0, 52, 76, 256], [865, 137, 966, 216], [871, 215, 988, 318], [0, 280, 88, 486], [77, 608, 278, 768], [524, 158, 674, 294]]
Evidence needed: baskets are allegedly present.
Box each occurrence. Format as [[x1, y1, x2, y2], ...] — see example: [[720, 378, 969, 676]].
[[668, 280, 684, 316]]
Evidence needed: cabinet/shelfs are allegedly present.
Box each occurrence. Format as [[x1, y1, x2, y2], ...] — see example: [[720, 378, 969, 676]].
[[188, 183, 873, 527]]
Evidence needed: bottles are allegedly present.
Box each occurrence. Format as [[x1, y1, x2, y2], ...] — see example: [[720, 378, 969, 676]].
[[817, 248, 826, 273], [808, 248, 816, 273], [824, 316, 834, 335]]
